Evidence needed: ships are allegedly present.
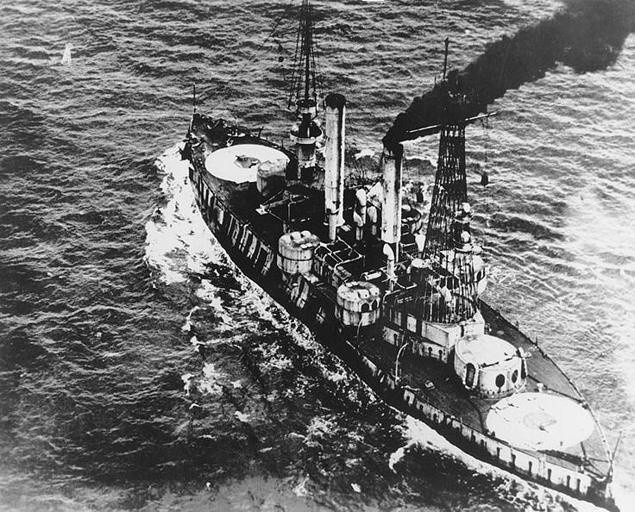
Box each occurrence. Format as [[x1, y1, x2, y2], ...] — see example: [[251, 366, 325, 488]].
[[178, 0, 629, 512]]
